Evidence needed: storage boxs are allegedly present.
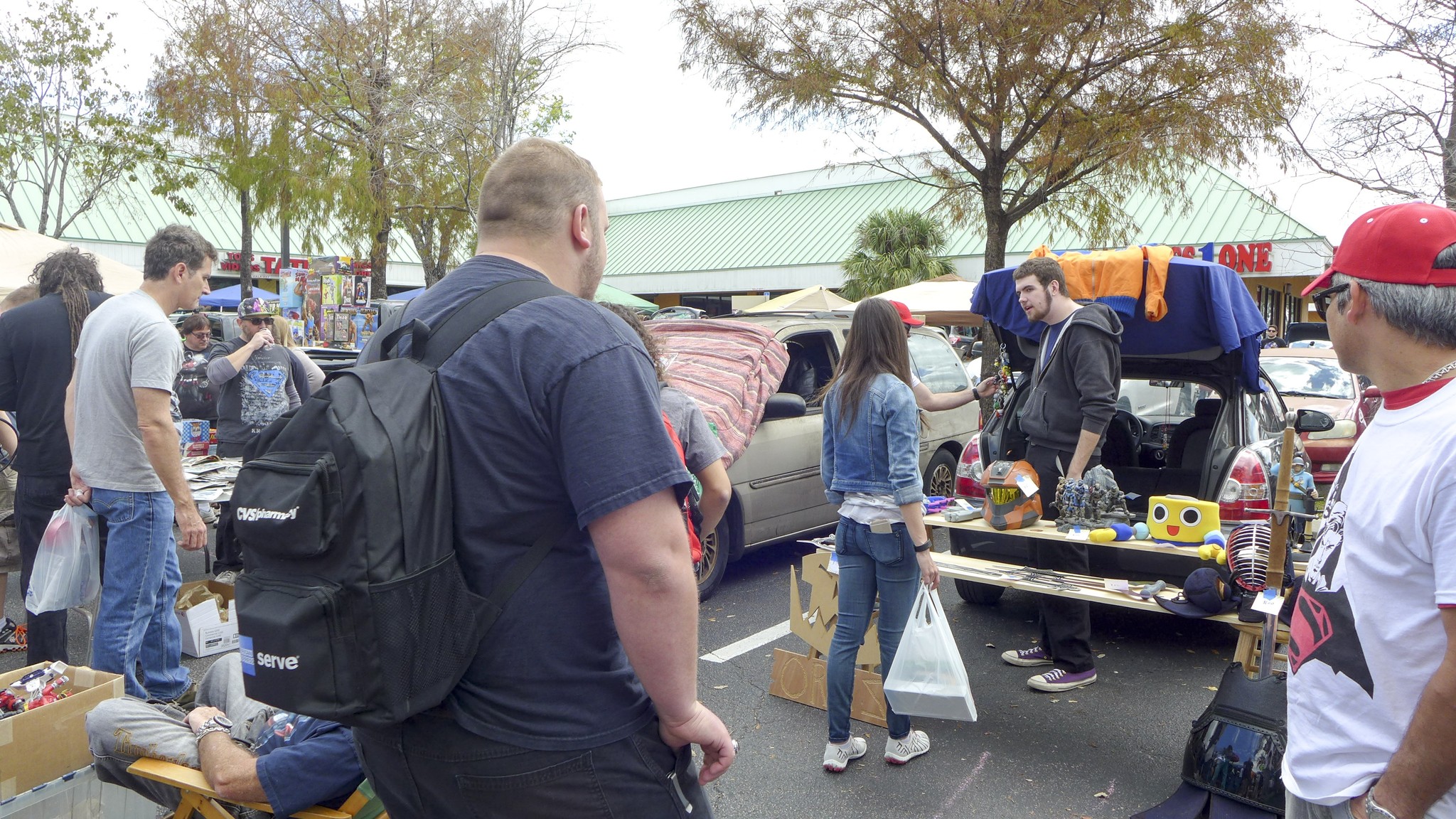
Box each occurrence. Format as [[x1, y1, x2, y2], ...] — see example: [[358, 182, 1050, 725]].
[[279, 255, 378, 349], [0, 661, 125, 819], [180, 417, 217, 456], [175, 579, 240, 657]]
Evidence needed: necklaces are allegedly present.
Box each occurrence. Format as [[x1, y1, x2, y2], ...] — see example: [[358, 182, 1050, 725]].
[[1421, 360, 1456, 383]]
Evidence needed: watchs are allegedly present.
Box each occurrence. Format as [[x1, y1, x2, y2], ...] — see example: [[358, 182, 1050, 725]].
[[196, 716, 233, 736], [1365, 785, 1398, 819], [913, 538, 933, 552]]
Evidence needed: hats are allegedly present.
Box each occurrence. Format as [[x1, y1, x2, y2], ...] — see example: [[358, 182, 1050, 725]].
[[237, 298, 276, 319], [1300, 200, 1456, 298], [888, 299, 924, 328]]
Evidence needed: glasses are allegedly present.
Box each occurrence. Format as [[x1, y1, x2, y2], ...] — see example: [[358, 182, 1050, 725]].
[[190, 332, 213, 339], [904, 323, 911, 333], [1311, 283, 1348, 323], [242, 317, 275, 325], [1267, 330, 1276, 333]]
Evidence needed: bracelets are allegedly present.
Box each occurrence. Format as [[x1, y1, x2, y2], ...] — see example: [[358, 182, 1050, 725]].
[[196, 727, 232, 745], [971, 386, 981, 401]]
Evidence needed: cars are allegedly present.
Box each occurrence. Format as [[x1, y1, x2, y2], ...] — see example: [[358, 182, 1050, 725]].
[[679, 307, 982, 600], [950, 321, 1383, 624], [643, 306, 707, 321], [176, 311, 241, 342]]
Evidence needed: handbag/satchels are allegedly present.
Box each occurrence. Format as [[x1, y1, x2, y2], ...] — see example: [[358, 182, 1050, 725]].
[[24, 489, 99, 616], [882, 579, 979, 723]]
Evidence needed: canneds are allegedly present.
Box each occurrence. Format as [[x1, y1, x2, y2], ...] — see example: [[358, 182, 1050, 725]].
[[259, 328, 275, 351]]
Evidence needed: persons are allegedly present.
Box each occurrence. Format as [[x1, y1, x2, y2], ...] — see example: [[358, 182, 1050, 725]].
[[172, 314, 221, 429], [1281, 202, 1456, 819], [63, 223, 219, 710], [1187, 741, 1284, 806], [821, 297, 1001, 772], [1269, 457, 1318, 548], [206, 297, 302, 458], [268, 315, 326, 405], [85, 652, 367, 819], [1260, 324, 1289, 349], [0, 246, 115, 666], [1001, 257, 1124, 693], [338, 134, 739, 818]]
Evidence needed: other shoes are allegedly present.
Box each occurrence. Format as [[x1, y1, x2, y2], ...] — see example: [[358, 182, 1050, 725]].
[[168, 683, 199, 709], [212, 515, 220, 527], [199, 506, 216, 523]]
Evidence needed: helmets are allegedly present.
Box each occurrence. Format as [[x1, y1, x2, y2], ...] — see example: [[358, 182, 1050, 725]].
[[1224, 524, 1286, 592], [979, 459, 1044, 531]]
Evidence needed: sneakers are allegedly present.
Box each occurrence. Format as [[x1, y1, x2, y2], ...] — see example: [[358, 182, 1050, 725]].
[[1001, 646, 1054, 666], [1026, 668, 1097, 692], [0, 617, 28, 653], [215, 571, 242, 585], [883, 730, 930, 765], [823, 735, 867, 772]]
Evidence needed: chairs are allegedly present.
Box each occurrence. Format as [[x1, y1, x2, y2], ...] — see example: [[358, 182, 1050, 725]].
[[126, 758, 389, 819], [1168, 398, 1223, 470]]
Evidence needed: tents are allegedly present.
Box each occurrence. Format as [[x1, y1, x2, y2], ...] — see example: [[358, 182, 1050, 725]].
[[198, 283, 280, 313], [387, 286, 428, 301]]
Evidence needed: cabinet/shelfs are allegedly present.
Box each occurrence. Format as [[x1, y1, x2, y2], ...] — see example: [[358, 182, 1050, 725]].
[[800, 494, 1265, 735]]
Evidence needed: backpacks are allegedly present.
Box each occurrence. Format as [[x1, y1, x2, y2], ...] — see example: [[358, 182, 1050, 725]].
[[225, 277, 574, 728]]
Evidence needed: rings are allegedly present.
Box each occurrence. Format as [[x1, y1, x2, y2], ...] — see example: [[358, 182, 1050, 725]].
[[731, 738, 741, 756]]
